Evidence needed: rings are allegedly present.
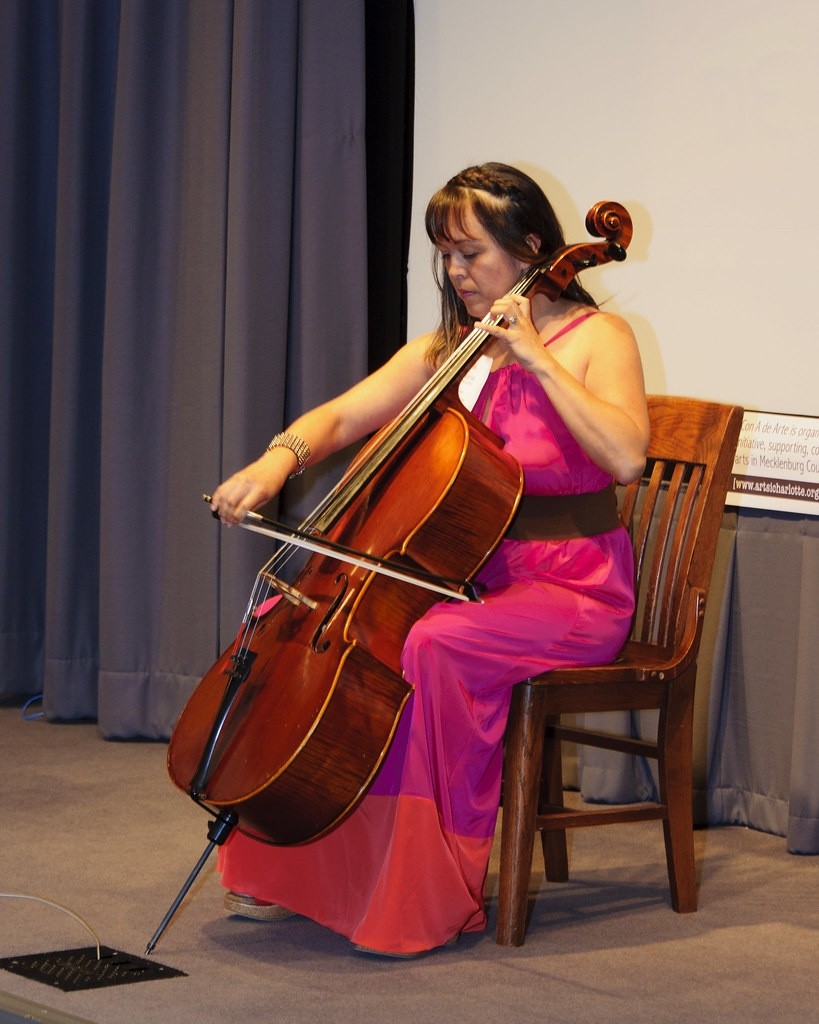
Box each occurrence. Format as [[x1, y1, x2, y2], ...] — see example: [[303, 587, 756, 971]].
[[511, 317, 520, 326]]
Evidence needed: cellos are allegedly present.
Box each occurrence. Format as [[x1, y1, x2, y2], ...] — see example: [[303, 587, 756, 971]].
[[145, 199, 632, 952]]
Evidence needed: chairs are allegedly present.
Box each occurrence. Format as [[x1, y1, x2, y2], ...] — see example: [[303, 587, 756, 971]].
[[492, 395, 744, 945]]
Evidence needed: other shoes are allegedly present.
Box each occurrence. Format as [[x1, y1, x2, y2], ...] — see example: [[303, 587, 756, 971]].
[[354, 936, 459, 958], [223, 890, 300, 921]]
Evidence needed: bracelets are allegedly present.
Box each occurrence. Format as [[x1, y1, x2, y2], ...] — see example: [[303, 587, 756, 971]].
[[266, 433, 312, 480]]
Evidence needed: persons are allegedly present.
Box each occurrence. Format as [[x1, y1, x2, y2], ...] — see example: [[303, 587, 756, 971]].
[[205, 161, 652, 958]]
